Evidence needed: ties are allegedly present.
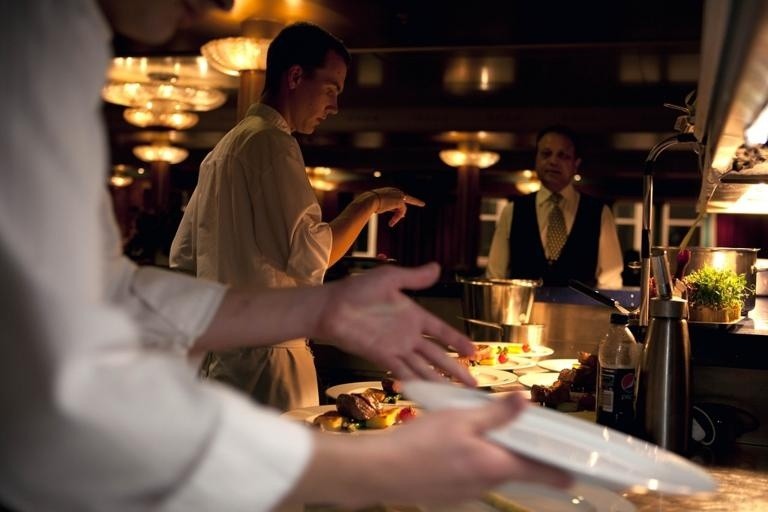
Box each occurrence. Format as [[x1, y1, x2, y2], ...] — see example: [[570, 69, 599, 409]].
[[546, 192, 567, 263]]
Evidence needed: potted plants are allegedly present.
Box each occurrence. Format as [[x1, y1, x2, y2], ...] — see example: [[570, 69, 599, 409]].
[[723, 268, 759, 323], [683, 264, 731, 323]]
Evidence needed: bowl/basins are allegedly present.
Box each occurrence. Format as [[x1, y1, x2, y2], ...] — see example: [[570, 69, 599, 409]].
[[455, 275, 542, 325]]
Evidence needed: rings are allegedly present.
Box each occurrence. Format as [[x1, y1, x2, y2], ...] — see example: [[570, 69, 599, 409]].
[[399, 190, 408, 200]]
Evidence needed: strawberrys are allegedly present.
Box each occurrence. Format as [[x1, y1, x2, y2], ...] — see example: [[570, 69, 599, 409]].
[[522, 344, 529, 352], [498, 352, 508, 363], [399, 404, 416, 422]]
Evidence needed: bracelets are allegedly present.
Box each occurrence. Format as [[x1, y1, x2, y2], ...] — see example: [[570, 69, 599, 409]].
[[367, 189, 381, 214]]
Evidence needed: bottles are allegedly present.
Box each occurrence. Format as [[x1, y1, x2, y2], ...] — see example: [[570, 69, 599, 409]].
[[596, 313, 638, 434], [639, 289, 692, 458]]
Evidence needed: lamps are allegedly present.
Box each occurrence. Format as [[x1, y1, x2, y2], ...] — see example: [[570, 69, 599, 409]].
[[98, 34, 277, 172]]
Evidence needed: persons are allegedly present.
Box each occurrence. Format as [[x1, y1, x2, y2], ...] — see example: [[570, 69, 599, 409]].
[[169, 19, 427, 417], [484, 123, 624, 290], [0, 0, 572, 512]]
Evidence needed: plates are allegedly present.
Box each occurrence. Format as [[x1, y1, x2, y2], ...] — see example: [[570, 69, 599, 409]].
[[326, 380, 401, 400], [280, 403, 424, 433], [425, 340, 583, 387]]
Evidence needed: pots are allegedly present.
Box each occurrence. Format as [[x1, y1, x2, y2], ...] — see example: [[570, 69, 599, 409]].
[[648, 240, 759, 316], [453, 315, 545, 345]]
